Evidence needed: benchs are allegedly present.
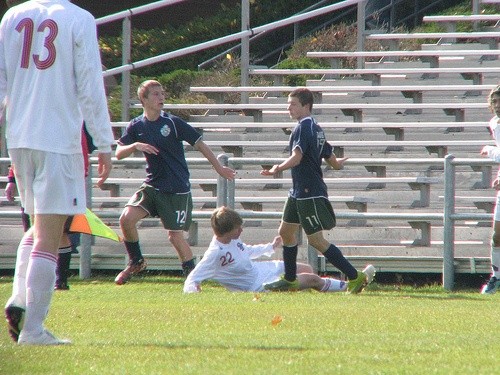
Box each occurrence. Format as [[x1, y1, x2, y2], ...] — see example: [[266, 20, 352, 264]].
[[1, 0, 499, 280]]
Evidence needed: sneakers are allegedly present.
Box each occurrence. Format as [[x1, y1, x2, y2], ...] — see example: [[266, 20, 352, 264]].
[[2, 298, 77, 348], [478, 277, 500, 296], [363, 264, 376, 286], [345, 271, 366, 294], [54, 270, 70, 291], [116, 261, 148, 286], [264, 276, 305, 294]]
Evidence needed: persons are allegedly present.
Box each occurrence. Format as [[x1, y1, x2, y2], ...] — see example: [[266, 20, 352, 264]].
[[183, 206, 376, 293], [260, 88, 369, 294], [114, 80, 236, 285], [480, 85, 500, 294], [0, 0, 115, 346]]
